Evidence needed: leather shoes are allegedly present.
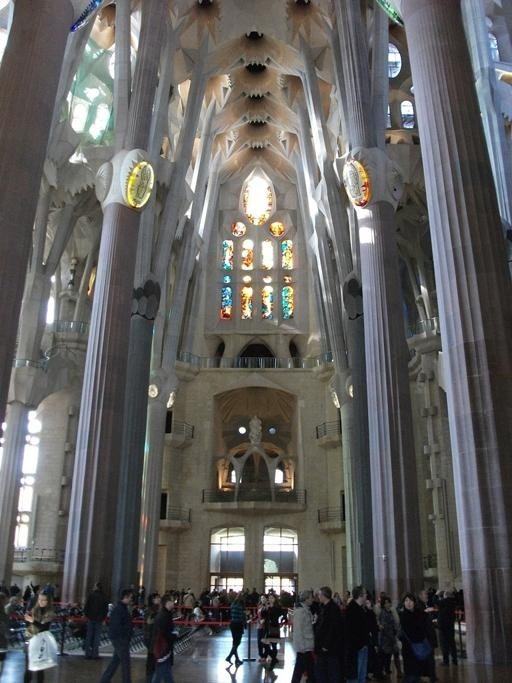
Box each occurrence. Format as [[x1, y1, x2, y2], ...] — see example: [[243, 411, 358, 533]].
[[226, 658, 233, 664], [235, 659, 242, 664]]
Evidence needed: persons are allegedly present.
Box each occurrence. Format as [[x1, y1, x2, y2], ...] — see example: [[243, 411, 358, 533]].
[[291, 586, 465, 683], [193, 587, 288, 668], [85, 583, 196, 683], [0, 583, 61, 683]]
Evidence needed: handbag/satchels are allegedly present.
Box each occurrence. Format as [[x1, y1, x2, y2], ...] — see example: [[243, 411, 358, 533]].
[[152, 634, 172, 663], [409, 639, 432, 660], [25, 631, 60, 672]]
